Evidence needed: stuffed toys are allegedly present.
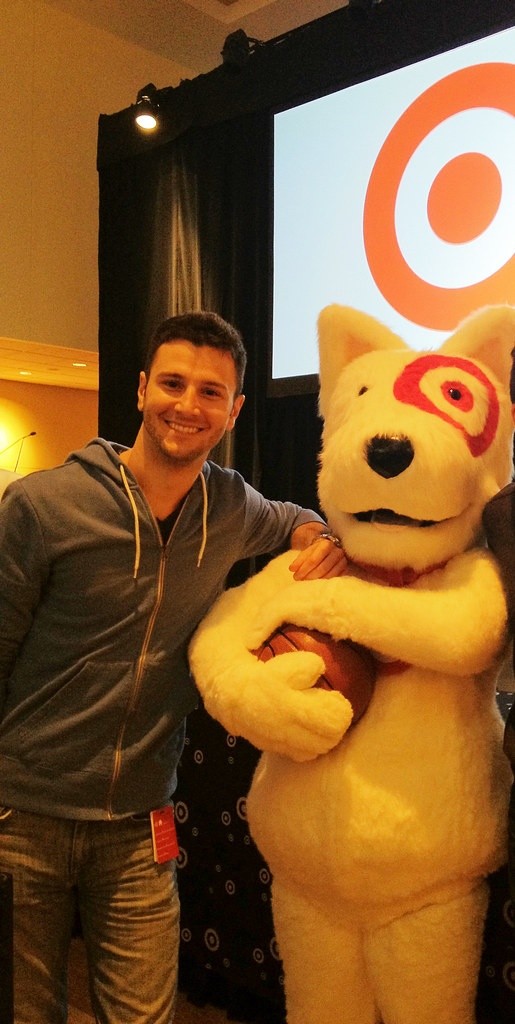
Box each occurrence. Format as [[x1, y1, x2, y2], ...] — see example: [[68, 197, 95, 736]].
[[184, 297, 515, 1024]]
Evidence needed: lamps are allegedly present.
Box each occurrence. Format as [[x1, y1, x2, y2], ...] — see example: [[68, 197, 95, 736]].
[[135, 82, 160, 131], [220, 29, 264, 79]]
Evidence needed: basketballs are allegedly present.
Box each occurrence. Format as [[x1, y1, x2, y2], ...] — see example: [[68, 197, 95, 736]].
[[254, 622, 376, 727]]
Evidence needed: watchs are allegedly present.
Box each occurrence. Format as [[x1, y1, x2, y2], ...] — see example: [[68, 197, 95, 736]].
[[311, 532, 347, 549]]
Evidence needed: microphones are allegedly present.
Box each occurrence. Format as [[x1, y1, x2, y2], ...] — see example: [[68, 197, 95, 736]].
[[0, 432, 36, 455]]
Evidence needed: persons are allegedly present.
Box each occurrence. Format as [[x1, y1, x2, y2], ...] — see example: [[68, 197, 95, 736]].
[[2, 304, 350, 1024]]
